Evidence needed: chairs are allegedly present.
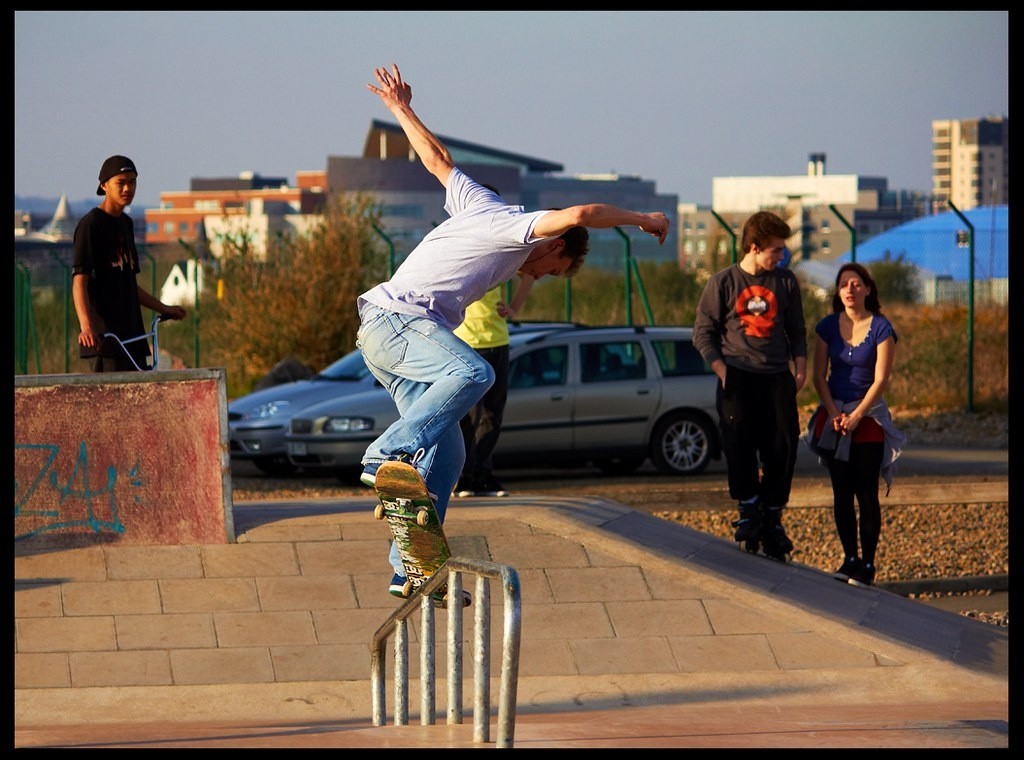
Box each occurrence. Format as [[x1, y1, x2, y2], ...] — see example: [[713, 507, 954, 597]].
[[604, 352, 621, 375]]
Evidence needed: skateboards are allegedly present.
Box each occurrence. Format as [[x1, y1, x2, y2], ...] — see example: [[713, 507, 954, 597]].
[[372, 461, 468, 610]]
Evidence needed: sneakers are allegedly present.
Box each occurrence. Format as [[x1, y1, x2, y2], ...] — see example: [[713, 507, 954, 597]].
[[848, 566, 875, 590], [833, 556, 862, 581], [360, 448, 439, 503], [388, 572, 471, 609]]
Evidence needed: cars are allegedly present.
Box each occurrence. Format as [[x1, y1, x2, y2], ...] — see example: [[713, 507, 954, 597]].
[[225, 317, 647, 481]]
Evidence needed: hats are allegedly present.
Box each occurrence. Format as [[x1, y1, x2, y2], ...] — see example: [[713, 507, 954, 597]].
[[96, 155, 137, 196]]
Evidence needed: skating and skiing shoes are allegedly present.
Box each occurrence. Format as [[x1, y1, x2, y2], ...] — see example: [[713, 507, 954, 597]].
[[731, 495, 760, 554], [758, 504, 793, 565]]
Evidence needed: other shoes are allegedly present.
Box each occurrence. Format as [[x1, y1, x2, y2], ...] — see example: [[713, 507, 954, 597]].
[[483, 482, 510, 497], [451, 480, 475, 498]]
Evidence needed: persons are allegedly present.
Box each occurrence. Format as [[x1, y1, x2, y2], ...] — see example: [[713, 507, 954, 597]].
[[813, 263, 899, 588], [692, 209, 810, 563], [351, 63, 670, 608], [69, 154, 187, 371], [452, 183, 536, 500]]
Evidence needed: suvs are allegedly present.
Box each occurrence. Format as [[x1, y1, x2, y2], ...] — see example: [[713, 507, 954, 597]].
[[279, 323, 725, 478]]
